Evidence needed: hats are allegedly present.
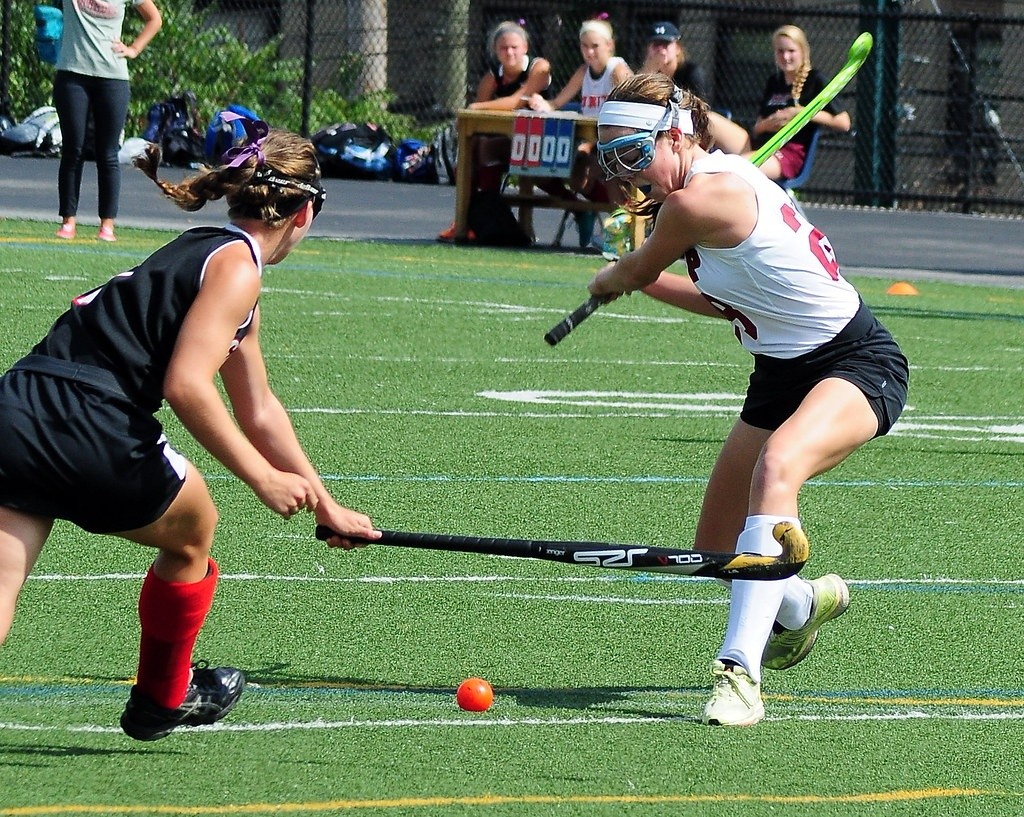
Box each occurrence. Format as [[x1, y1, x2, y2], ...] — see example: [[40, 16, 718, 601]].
[[646, 21, 681, 42]]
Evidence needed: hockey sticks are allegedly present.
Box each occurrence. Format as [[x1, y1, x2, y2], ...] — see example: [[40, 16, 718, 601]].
[[540, 27, 882, 351], [311, 517, 814, 586]]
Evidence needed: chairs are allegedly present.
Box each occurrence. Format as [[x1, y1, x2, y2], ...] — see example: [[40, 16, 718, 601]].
[[778, 127, 821, 223], [500, 102, 732, 249]]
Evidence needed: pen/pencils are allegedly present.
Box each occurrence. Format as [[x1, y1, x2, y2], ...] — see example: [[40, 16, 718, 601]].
[[520, 97, 528, 101]]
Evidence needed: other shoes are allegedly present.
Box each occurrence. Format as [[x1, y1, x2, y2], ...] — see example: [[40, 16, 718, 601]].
[[591, 212, 631, 261], [57, 224, 77, 240], [439, 223, 475, 244], [99, 226, 117, 241], [575, 209, 598, 248]]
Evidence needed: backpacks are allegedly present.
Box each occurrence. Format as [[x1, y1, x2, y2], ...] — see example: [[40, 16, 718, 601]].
[[204, 104, 268, 166], [394, 137, 440, 186], [435, 123, 458, 186], [22, 105, 62, 157], [144, 93, 206, 166]]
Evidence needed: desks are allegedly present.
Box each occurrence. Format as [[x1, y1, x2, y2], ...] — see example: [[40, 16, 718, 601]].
[[454, 107, 648, 254]]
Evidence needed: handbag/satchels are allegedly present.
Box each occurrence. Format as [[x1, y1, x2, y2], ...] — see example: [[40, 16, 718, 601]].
[[33, 6, 64, 65], [310, 123, 397, 182]]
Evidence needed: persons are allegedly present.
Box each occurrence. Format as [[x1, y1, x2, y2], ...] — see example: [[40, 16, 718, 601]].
[[0, 111, 382, 742], [437, 14, 851, 244], [50, 1, 162, 242], [589, 74, 909, 730]]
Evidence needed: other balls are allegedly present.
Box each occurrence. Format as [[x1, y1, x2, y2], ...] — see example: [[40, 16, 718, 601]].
[[455, 675, 497, 715]]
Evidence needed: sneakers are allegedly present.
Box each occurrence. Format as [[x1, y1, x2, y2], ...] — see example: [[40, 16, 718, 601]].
[[120, 660, 246, 741], [762, 574, 850, 669], [701, 660, 765, 725]]
